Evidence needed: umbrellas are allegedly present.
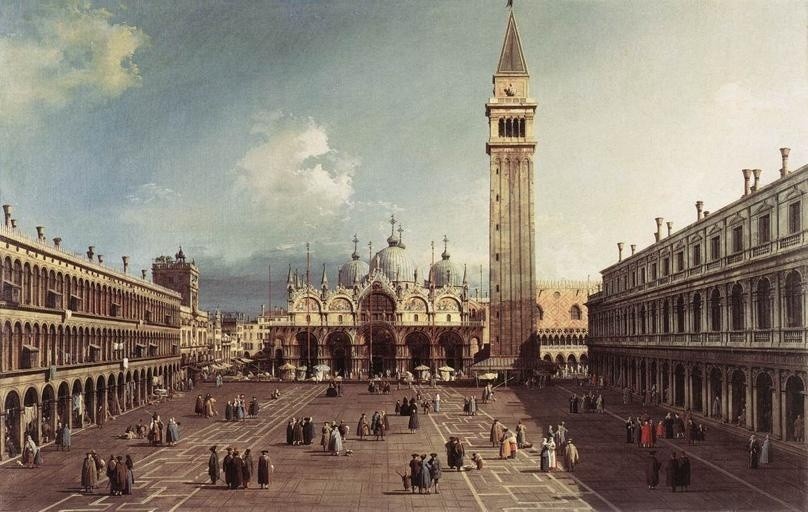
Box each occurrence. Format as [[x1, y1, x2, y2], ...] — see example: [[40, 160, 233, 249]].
[[278, 363, 296, 370], [413, 364, 431, 370]]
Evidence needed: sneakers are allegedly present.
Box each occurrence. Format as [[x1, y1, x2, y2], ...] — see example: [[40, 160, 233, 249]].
[[450, 466, 462, 472], [210, 481, 269, 490], [462, 412, 476, 416], [83, 488, 133, 496], [647, 486, 692, 493], [147, 443, 177, 447], [227, 416, 259, 423], [628, 436, 701, 448], [290, 438, 347, 457], [57, 447, 70, 453], [356, 434, 386, 441], [410, 432, 416, 434], [411, 485, 440, 494]]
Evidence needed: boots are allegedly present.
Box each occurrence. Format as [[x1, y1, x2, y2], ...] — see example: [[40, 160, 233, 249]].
[[518, 443, 524, 449]]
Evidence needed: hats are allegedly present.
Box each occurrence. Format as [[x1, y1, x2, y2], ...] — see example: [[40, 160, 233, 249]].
[[209, 445, 269, 455]]
